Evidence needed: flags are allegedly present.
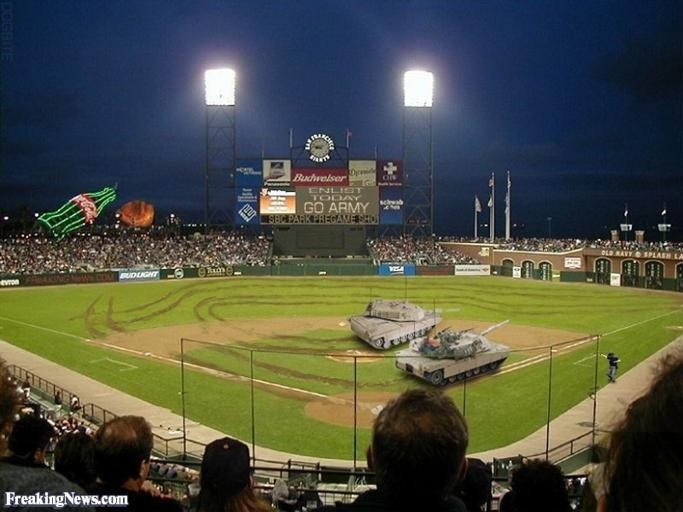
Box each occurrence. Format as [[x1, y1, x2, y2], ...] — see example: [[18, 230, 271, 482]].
[[476, 198, 482, 213]]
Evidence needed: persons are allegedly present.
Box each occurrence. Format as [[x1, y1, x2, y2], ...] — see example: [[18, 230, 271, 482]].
[[601, 352, 621, 382], [597, 355, 683, 512], [0, 365, 617, 512], [369, 233, 683, 292], [0, 226, 282, 277]]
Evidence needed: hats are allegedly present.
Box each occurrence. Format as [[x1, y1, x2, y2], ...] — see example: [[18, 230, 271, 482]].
[[200, 437, 250, 498]]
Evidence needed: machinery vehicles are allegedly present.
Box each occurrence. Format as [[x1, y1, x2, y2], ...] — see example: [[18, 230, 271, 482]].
[[347, 274, 510, 387]]
